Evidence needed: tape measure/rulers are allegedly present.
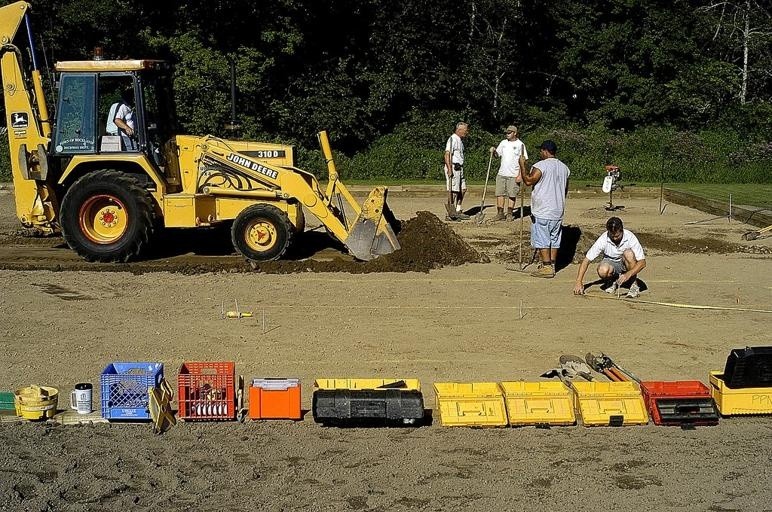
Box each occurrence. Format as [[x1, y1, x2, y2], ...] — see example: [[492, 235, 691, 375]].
[[582, 294, 772, 312]]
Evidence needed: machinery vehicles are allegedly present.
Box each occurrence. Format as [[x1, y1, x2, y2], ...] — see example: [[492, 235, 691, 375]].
[[0, 2, 400, 265]]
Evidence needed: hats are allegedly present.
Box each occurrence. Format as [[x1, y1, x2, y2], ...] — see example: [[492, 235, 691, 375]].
[[503, 125, 518, 135]]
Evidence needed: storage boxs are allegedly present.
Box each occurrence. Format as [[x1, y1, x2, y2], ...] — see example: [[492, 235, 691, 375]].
[[100, 346, 772, 427]]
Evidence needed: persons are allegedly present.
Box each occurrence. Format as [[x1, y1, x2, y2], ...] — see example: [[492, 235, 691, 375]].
[[571, 216, 646, 299], [443, 121, 471, 221], [518, 138, 571, 279], [489, 124, 528, 222], [113, 86, 139, 153]]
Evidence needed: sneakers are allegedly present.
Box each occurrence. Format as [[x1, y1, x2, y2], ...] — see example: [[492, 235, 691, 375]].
[[506, 212, 513, 222], [530, 265, 557, 279], [489, 212, 505, 222], [626, 283, 640, 298], [445, 211, 471, 222], [606, 281, 618, 294]]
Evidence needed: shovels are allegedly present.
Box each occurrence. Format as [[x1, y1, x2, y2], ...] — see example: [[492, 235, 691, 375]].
[[475, 147, 494, 224], [542, 351, 630, 382], [445, 137, 457, 219]]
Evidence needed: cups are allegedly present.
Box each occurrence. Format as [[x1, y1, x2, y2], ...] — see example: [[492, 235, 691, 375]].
[[70, 383, 93, 415]]
